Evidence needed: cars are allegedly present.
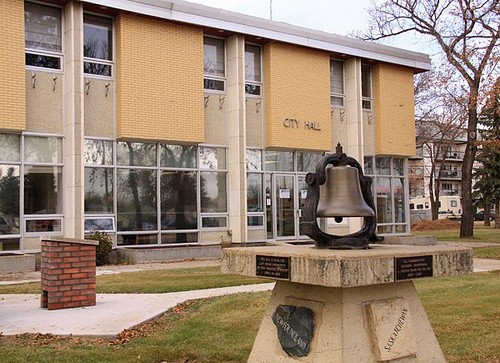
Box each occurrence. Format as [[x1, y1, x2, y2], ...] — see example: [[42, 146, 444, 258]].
[[472, 210, 496, 222]]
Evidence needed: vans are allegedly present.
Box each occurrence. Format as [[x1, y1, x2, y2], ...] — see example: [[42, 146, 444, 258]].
[[408, 195, 461, 220]]
